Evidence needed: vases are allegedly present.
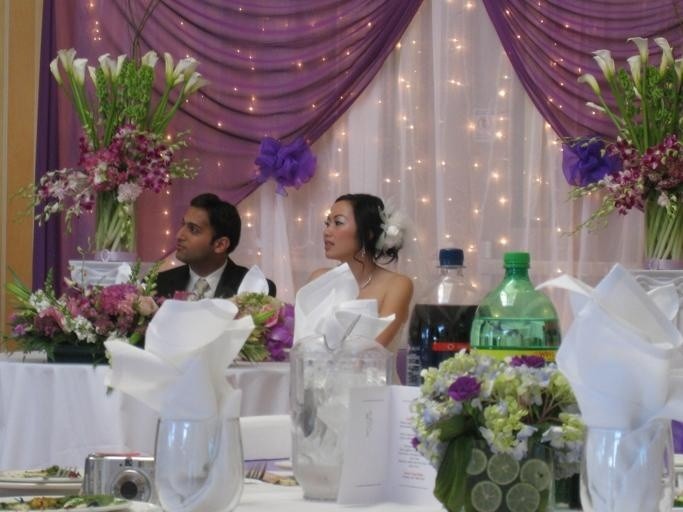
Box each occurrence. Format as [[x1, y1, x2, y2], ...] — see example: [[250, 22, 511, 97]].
[[92, 180, 139, 264], [464, 439, 558, 511], [44, 331, 106, 365]]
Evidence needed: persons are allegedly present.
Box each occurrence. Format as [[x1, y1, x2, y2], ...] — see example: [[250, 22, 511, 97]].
[[143, 194, 276, 299], [307, 193, 414, 384]]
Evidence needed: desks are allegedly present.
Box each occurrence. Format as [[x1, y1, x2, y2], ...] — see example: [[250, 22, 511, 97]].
[[0, 349, 291, 472]]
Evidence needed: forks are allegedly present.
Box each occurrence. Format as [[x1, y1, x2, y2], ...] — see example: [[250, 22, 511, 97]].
[[245, 461, 268, 481]]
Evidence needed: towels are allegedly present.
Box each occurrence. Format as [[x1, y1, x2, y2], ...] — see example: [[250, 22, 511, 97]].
[[535, 268, 682, 511], [294, 262, 396, 349], [236, 264, 269, 296], [101, 296, 254, 509]]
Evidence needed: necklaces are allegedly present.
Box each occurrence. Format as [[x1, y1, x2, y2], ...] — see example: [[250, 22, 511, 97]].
[[361, 275, 373, 288]]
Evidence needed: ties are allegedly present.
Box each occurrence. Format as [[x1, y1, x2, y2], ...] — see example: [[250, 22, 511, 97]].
[[189, 278, 209, 302]]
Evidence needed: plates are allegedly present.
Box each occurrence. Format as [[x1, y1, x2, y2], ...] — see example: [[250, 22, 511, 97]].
[[0, 477, 84, 495], [0, 495, 162, 512]]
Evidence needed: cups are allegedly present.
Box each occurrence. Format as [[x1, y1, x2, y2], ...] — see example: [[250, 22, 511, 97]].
[[575, 428, 678, 510], [153, 417, 244, 512], [288, 349, 395, 505]]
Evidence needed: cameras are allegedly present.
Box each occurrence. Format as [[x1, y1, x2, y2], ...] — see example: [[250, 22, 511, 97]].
[[83, 455, 157, 503]]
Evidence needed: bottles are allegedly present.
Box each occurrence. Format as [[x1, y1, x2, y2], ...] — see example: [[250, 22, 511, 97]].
[[469, 251, 559, 389], [402, 246, 485, 391]]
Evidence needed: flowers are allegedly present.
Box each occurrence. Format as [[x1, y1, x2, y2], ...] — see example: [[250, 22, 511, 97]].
[[561, 29, 682, 269], [228, 290, 296, 364], [0, 266, 194, 364], [403, 356, 585, 471], [6, 46, 213, 253]]
